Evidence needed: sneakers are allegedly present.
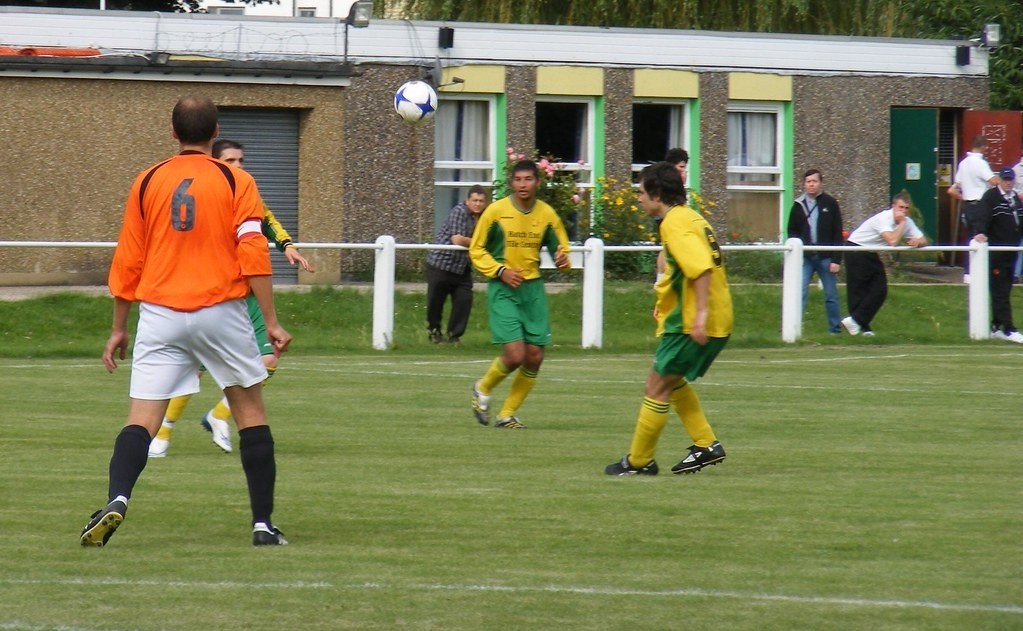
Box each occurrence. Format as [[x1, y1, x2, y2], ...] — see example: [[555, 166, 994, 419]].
[[253, 530, 288, 546], [863, 331, 874, 335], [81, 500, 126, 547], [471, 381, 490, 426], [1007, 332, 1023, 343], [605, 454, 660, 475], [841, 317, 860, 335], [493, 415, 527, 429], [147, 436, 169, 457], [991, 330, 1009, 340], [671, 440, 726, 474], [200, 408, 232, 453]]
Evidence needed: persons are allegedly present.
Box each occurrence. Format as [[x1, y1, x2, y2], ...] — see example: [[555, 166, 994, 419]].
[[946, 134, 1000, 235], [841, 190, 928, 336], [972, 155, 1023, 344], [652, 146, 688, 225], [146, 140, 309, 458], [788, 168, 844, 335], [425, 184, 488, 345], [469, 159, 572, 428], [81, 94, 290, 548], [604, 162, 734, 472]]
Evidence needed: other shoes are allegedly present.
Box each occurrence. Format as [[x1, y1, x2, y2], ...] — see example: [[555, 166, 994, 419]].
[[452, 340, 462, 348], [429, 333, 443, 344]]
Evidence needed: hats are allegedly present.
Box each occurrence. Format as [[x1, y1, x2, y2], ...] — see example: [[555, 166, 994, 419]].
[[1000, 168, 1015, 179]]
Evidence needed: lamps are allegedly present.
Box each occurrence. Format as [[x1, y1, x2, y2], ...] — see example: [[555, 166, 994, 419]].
[[979, 21, 1000, 48], [342, 2, 374, 27]]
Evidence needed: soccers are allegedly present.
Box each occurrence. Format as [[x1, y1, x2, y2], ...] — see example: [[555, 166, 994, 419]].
[[393, 80, 437, 125]]
